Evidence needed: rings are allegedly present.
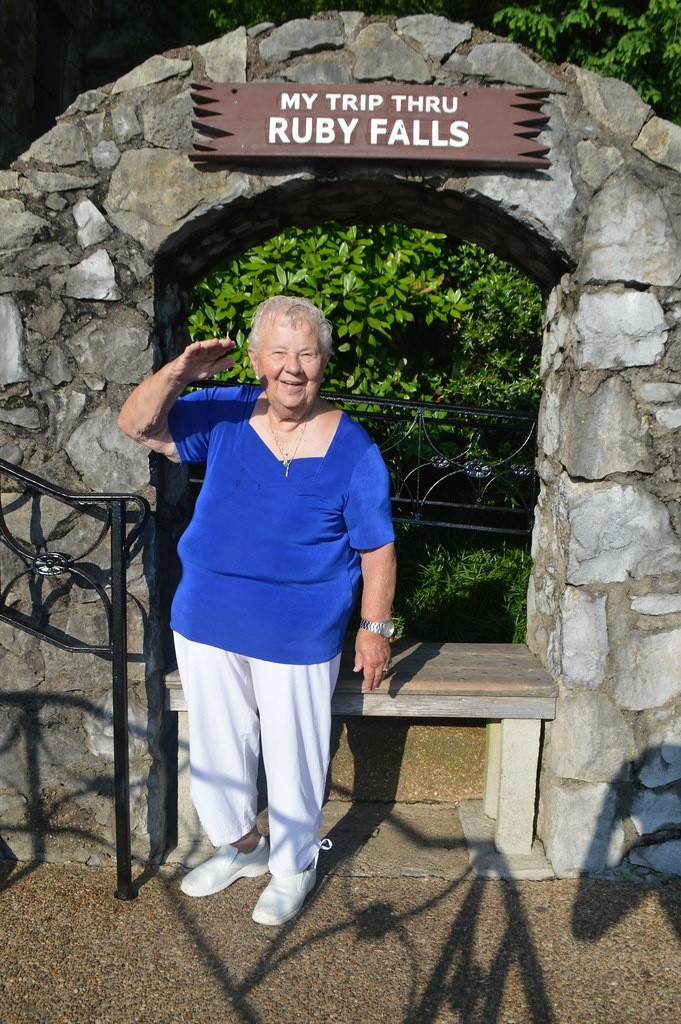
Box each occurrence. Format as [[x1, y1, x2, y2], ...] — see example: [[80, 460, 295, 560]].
[[383, 668, 389, 672]]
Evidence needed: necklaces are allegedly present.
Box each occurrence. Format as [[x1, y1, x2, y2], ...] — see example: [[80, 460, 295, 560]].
[[268, 412, 307, 478]]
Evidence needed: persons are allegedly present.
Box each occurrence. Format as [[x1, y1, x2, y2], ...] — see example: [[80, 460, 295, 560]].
[[117, 291, 398, 924]]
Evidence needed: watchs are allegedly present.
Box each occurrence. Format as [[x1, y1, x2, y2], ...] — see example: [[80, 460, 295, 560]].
[[359, 619, 395, 638]]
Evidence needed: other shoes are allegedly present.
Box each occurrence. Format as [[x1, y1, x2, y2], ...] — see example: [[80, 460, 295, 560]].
[[252, 851, 318, 925], [180, 834, 270, 898]]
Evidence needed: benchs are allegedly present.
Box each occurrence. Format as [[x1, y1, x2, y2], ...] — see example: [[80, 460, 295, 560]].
[[163, 641, 559, 855]]
